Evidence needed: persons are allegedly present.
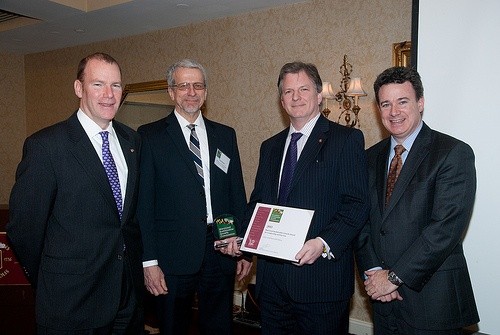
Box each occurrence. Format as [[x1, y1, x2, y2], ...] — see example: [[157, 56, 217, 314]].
[[220, 62, 371, 335], [6, 53, 141, 335], [134, 59, 254, 335], [354, 66, 481, 335]]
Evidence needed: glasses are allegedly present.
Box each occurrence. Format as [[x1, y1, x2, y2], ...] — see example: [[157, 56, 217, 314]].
[[172, 82, 205, 91]]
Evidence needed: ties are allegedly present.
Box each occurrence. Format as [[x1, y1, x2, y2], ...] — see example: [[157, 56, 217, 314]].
[[186, 124, 204, 186], [276, 132, 303, 205], [385, 145, 406, 207], [99, 131, 123, 221]]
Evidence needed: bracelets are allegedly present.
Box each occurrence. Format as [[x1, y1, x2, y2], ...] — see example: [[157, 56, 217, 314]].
[[322, 243, 328, 259]]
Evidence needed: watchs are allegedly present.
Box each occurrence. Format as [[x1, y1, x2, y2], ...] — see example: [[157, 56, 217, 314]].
[[387, 270, 402, 287]]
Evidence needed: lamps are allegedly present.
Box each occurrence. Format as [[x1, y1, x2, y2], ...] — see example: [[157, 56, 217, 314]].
[[319, 55, 367, 131]]
